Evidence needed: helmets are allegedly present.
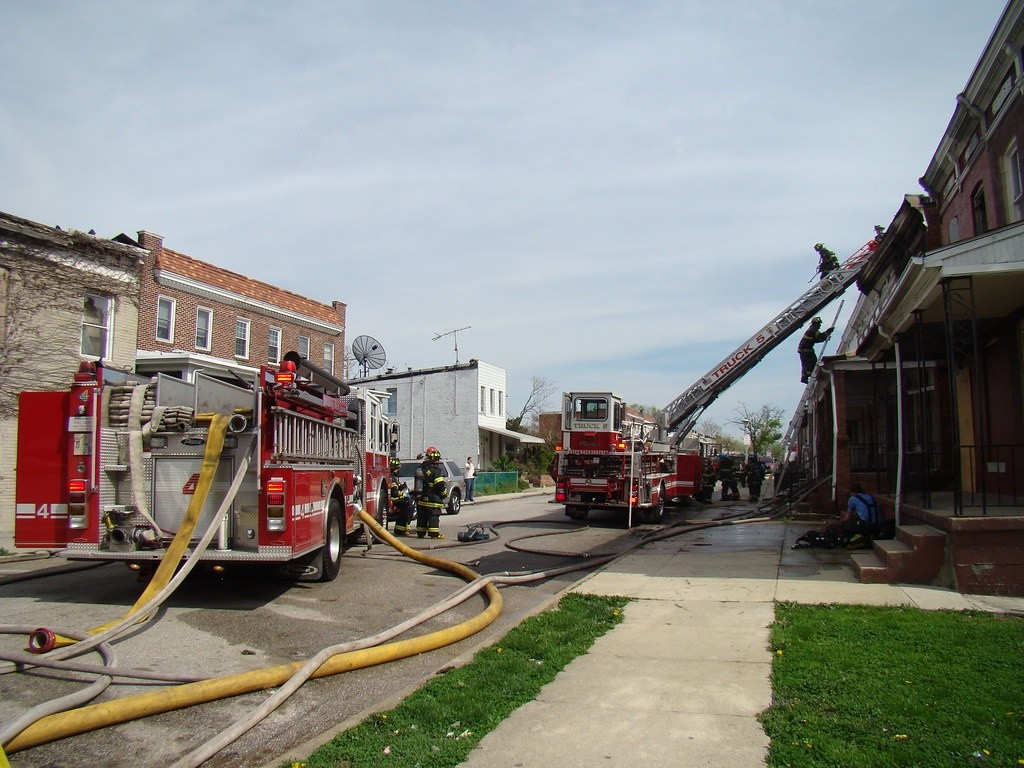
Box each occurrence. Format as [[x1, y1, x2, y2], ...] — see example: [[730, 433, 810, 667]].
[[428, 450, 442, 460], [390, 457, 401, 469], [747, 455, 755, 462], [425, 447, 437, 455], [719, 453, 729, 458]]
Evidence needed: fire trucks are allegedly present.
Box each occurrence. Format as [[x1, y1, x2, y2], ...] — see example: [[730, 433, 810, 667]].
[[13, 350, 400, 583], [546, 240, 878, 524]]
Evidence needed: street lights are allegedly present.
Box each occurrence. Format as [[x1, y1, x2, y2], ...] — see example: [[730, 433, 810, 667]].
[[741, 417, 750, 465]]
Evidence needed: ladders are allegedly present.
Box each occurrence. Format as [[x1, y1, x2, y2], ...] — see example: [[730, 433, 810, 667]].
[[272, 407, 359, 466], [655, 240, 884, 419], [757, 298, 845, 497]]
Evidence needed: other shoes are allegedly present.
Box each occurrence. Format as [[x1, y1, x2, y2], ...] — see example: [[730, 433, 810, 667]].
[[431, 534, 445, 540], [847, 541, 865, 549], [417, 534, 425, 539]]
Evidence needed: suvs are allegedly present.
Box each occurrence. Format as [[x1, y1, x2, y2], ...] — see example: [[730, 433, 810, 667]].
[[391, 458, 465, 515]]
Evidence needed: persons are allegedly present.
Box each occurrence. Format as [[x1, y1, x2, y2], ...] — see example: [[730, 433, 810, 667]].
[[797, 317, 835, 383], [845, 485, 881, 542], [874, 225, 886, 240], [414, 447, 447, 538], [705, 453, 765, 503], [390, 458, 415, 536], [465, 457, 475, 502], [814, 242, 839, 278]]
